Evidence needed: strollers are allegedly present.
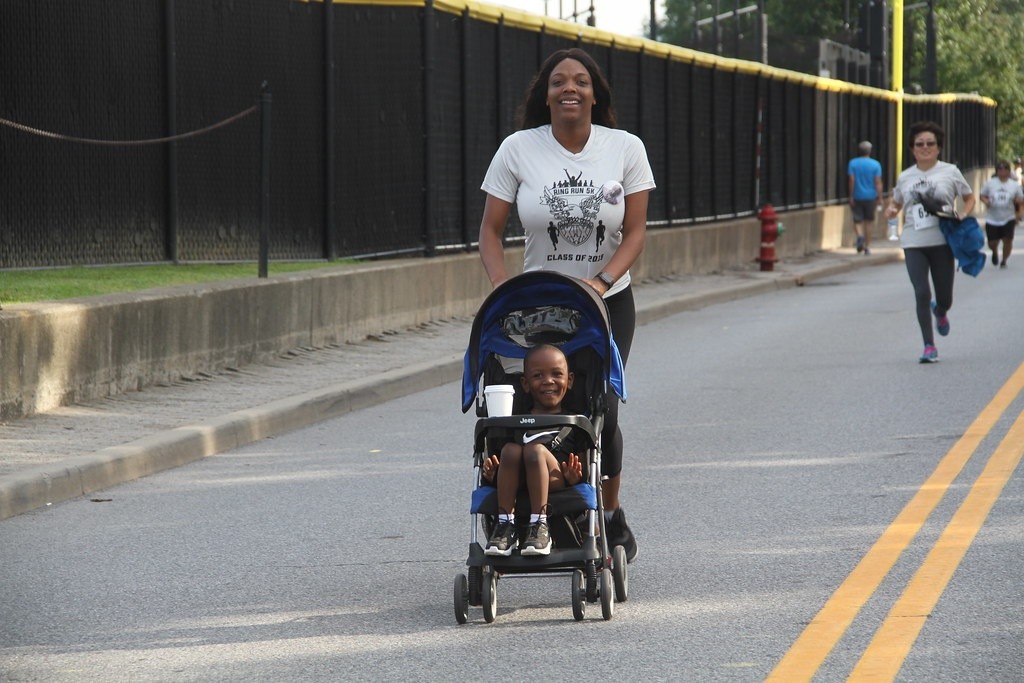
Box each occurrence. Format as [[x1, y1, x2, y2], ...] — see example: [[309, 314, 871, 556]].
[[449, 268, 629, 627]]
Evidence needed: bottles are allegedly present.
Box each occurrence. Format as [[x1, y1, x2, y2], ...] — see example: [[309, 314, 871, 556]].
[[886, 208, 899, 243]]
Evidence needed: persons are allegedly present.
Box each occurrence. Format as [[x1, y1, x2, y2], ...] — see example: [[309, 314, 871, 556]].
[[482, 342, 587, 557], [883, 123, 976, 363], [980, 159, 1024, 267], [848, 141, 883, 256], [478, 48, 656, 564]]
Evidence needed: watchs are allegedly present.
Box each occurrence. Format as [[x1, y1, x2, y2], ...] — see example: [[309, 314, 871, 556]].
[[594, 271, 615, 291]]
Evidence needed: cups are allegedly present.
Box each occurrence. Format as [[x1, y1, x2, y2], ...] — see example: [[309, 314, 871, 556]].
[[482, 384, 516, 417]]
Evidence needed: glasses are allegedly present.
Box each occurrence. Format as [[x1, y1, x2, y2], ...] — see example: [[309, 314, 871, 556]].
[[913, 142, 937, 146], [998, 166, 1009, 170]]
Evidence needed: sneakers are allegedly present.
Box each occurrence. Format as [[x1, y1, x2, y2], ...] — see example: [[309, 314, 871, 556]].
[[604, 508, 638, 563], [931, 301, 950, 336], [520, 504, 553, 555], [920, 345, 938, 362], [484, 506, 520, 556]]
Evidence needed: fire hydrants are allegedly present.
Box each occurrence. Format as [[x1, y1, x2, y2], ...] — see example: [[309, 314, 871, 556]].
[[756, 203, 785, 272]]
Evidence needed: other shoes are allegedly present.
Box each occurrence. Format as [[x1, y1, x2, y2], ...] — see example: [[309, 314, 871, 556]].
[[865, 249, 870, 255], [1002, 260, 1007, 268], [992, 249, 998, 265], [857, 240, 864, 253]]
